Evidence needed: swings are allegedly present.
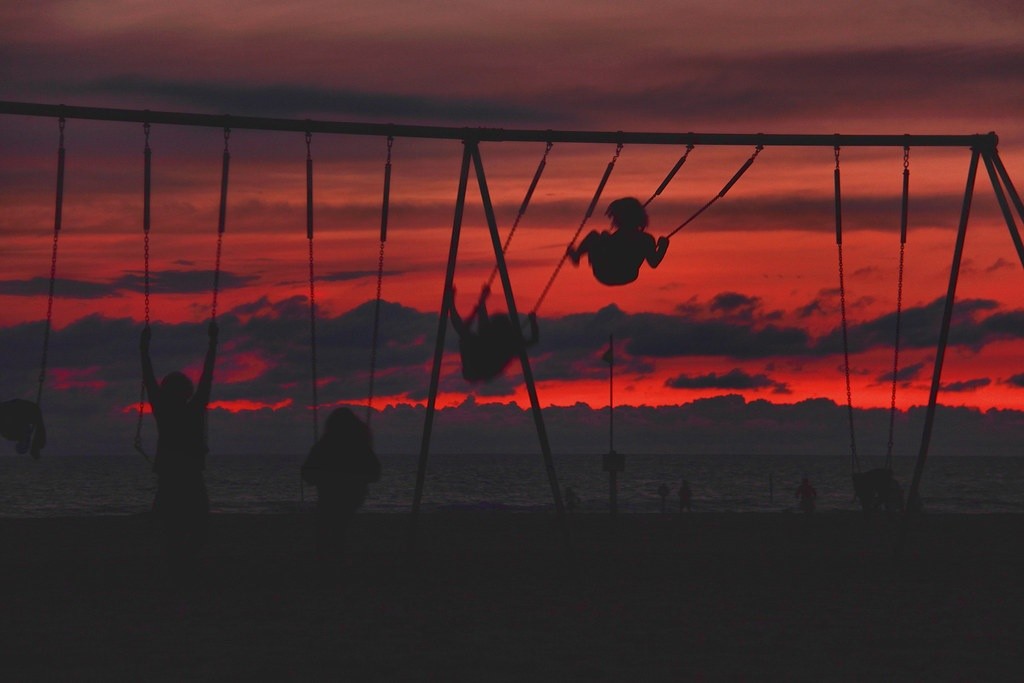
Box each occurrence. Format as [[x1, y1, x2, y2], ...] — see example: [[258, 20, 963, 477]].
[[459, 138, 624, 386], [297, 131, 398, 524], [825, 145, 921, 514], [0, 116, 72, 455], [585, 143, 767, 285], [122, 115, 226, 492]]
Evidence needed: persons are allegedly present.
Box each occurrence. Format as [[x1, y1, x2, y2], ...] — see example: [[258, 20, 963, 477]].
[[129, 316, 225, 579], [448, 280, 542, 385], [300, 404, 386, 580], [792, 475, 819, 512], [562, 194, 670, 287], [563, 488, 585, 517]]
